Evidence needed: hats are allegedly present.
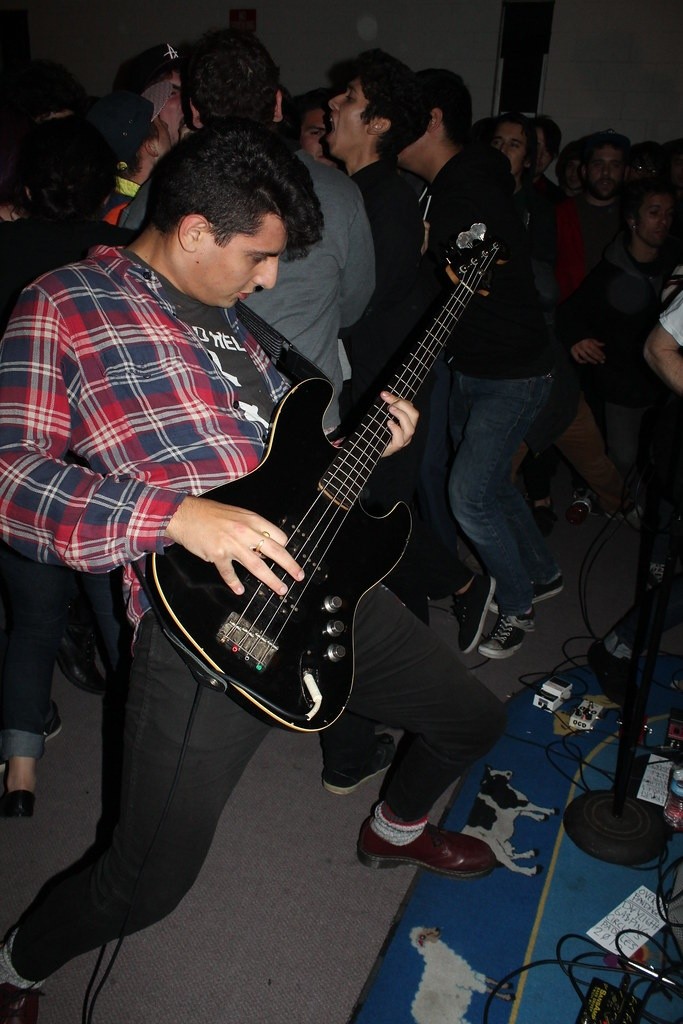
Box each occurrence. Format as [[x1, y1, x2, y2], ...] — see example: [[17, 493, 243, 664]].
[[80, 80, 173, 174], [117, 42, 186, 97]]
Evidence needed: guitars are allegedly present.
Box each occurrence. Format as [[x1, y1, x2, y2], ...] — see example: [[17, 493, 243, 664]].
[[151, 219, 503, 730]]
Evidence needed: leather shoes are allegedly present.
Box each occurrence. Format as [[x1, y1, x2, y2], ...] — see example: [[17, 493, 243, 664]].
[[57, 609, 106, 696], [0, 984, 44, 1024], [357, 820, 495, 878], [2, 790, 36, 820]]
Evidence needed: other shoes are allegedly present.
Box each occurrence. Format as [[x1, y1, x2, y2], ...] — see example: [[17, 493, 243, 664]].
[[534, 495, 558, 537], [0, 699, 62, 775]]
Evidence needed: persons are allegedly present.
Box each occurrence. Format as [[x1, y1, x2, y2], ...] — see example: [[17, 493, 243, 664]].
[[0, 31, 683, 817], [0, 124, 507, 1024]]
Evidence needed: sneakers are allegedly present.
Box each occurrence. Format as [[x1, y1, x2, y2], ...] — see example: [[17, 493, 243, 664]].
[[490, 569, 566, 616], [322, 732, 396, 795], [478, 614, 535, 659], [449, 571, 495, 653]]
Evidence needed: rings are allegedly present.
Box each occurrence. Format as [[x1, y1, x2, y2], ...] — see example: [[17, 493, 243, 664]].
[[256, 532, 271, 555]]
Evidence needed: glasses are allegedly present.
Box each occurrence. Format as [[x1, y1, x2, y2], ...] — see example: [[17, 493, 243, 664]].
[[628, 159, 660, 179]]
